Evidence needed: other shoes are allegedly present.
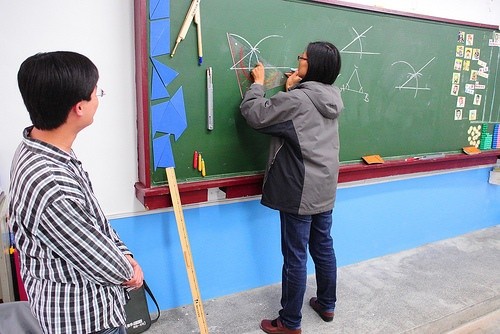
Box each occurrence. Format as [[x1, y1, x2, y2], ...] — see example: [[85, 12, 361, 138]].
[[309, 297, 335, 322], [260, 317, 302, 334]]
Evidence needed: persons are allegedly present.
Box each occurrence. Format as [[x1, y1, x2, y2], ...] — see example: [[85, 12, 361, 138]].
[[8, 51, 144, 334], [240, 41, 344, 334]]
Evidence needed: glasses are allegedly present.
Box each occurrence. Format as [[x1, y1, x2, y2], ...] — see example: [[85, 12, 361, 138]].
[[96, 87, 106, 98], [298, 55, 307, 62]]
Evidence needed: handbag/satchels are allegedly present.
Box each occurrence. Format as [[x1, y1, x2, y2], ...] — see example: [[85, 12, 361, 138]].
[[124, 279, 161, 334]]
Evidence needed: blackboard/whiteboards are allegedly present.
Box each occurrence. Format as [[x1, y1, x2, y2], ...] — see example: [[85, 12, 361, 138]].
[[134, 0, 500, 211]]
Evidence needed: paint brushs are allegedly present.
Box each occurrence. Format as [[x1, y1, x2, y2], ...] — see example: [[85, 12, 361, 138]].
[[290, 66, 297, 72], [193, 150, 207, 177]]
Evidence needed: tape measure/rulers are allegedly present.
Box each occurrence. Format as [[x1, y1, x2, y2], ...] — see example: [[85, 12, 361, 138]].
[[227, 32, 288, 99]]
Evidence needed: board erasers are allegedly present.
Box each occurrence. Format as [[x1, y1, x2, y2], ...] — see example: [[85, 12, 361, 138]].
[[361, 154, 385, 164], [463, 146, 482, 155]]
